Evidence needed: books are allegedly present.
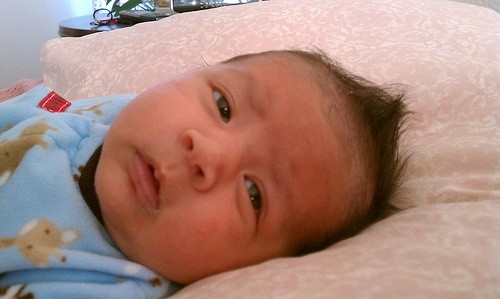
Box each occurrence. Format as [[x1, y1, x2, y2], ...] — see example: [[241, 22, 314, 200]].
[[119, 10, 175, 25]]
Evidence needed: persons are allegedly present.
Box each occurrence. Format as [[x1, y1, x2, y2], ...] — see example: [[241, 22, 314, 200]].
[[0, 50, 416, 299]]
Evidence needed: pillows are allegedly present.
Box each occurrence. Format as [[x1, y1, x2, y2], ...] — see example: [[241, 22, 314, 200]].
[[39, 0, 500, 299]]
